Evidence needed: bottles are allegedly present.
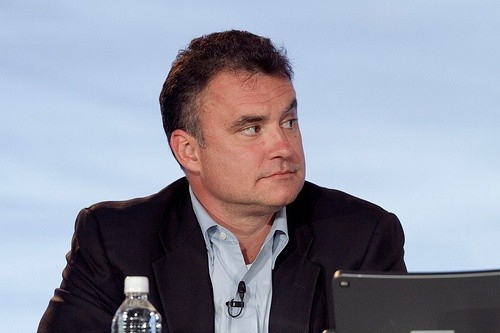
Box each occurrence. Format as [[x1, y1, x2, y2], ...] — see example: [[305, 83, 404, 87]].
[[110, 275, 162, 333]]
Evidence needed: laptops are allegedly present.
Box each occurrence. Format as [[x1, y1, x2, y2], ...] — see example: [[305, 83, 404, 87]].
[[331, 269, 500, 333]]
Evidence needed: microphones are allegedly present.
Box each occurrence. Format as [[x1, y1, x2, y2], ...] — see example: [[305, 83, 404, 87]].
[[237, 281, 245, 299]]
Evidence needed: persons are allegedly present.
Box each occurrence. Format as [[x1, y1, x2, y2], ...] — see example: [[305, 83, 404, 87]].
[[35, 29, 410, 333]]
